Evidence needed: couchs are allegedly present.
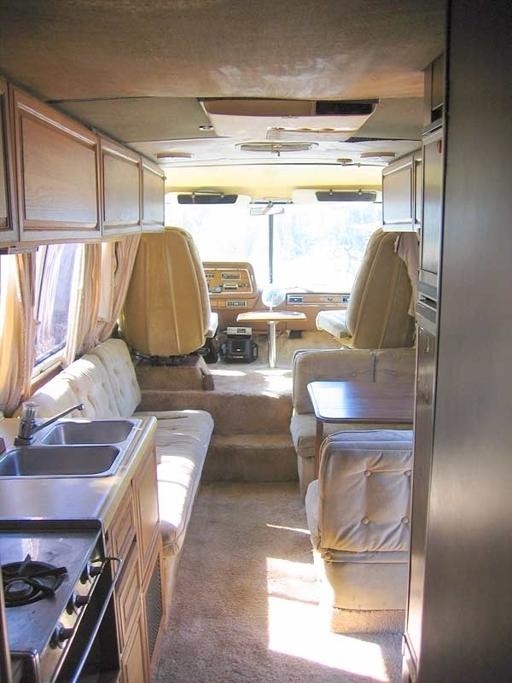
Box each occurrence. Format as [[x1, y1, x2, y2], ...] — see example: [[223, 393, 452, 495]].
[[11, 338, 215, 560], [290, 346, 417, 636]]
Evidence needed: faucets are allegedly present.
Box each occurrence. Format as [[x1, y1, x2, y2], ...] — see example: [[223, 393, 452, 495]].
[[14, 401, 85, 445]]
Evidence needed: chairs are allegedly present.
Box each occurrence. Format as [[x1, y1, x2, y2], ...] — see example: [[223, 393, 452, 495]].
[[315, 224, 420, 350], [110, 227, 222, 392]]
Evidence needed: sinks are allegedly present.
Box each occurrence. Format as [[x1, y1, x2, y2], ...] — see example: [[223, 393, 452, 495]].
[[34, 417, 143, 444], [0, 446, 123, 478]]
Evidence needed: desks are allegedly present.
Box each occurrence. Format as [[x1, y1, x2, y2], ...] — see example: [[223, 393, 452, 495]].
[[237, 311, 307, 368]]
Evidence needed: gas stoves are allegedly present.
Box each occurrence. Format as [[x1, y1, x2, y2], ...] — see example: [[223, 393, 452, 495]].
[[1, 527, 108, 683]]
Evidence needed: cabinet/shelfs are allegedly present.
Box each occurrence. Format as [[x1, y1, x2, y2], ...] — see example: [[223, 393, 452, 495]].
[[0, 77, 167, 251], [381, 149, 422, 233], [106, 438, 167, 683]]
